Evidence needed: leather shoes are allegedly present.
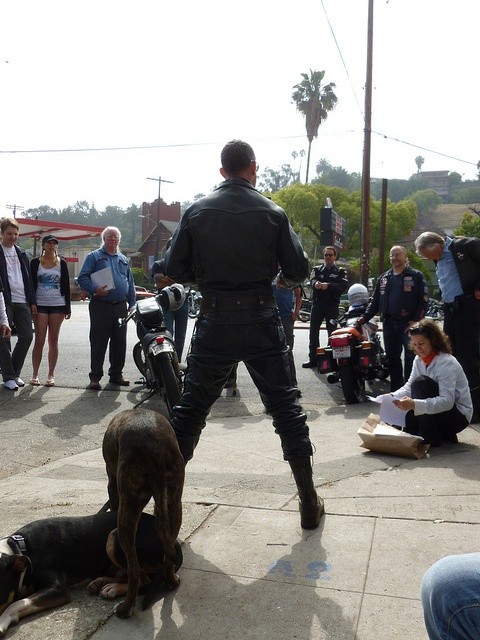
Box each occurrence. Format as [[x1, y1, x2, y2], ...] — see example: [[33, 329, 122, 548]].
[[302, 361, 317, 368], [109, 377, 130, 386], [90, 379, 99, 388]]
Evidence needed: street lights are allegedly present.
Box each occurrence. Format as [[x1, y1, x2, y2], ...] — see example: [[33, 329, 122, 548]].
[[137, 175, 176, 261]]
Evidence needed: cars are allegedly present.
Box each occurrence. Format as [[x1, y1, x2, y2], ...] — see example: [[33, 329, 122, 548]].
[[134, 286, 156, 300]]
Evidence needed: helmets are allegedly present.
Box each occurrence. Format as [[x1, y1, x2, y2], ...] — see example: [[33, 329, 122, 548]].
[[347, 283, 370, 307], [161, 282, 186, 312]]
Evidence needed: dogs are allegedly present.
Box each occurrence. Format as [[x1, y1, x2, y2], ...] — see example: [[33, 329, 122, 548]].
[[100, 407, 185, 619], [0, 511, 183, 637]]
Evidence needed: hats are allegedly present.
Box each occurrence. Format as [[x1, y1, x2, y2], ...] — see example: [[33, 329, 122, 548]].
[[42, 234, 59, 244]]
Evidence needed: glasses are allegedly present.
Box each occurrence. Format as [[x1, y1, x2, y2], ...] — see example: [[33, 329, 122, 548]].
[[251, 160, 260, 171], [324, 253, 334, 257]]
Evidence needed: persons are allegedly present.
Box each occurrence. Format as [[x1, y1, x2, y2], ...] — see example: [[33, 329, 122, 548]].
[[356, 245, 429, 392], [302, 246, 348, 368], [388, 318, 474, 447], [0, 217, 37, 391], [78, 226, 136, 389], [414, 231, 480, 424], [224, 363, 237, 389], [152, 237, 190, 363], [271, 263, 301, 387], [420, 552, 480, 640], [0, 276, 11, 338], [163, 140, 324, 529], [31, 235, 71, 386]]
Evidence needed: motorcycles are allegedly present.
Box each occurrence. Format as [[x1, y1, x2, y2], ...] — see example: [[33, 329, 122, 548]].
[[154, 286, 202, 318], [425, 298, 445, 318], [327, 294, 390, 404], [298, 298, 346, 323], [117, 282, 188, 420]]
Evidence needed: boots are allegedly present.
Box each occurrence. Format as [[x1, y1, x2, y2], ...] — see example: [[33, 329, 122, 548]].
[[289, 455, 324, 530]]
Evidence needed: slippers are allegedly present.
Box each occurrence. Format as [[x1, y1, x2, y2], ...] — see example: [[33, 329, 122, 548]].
[[30, 377, 40, 385], [44, 379, 54, 386]]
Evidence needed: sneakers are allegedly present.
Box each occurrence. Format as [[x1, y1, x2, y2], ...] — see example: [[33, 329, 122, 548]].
[[4, 379, 18, 391], [15, 377, 25, 387]]
